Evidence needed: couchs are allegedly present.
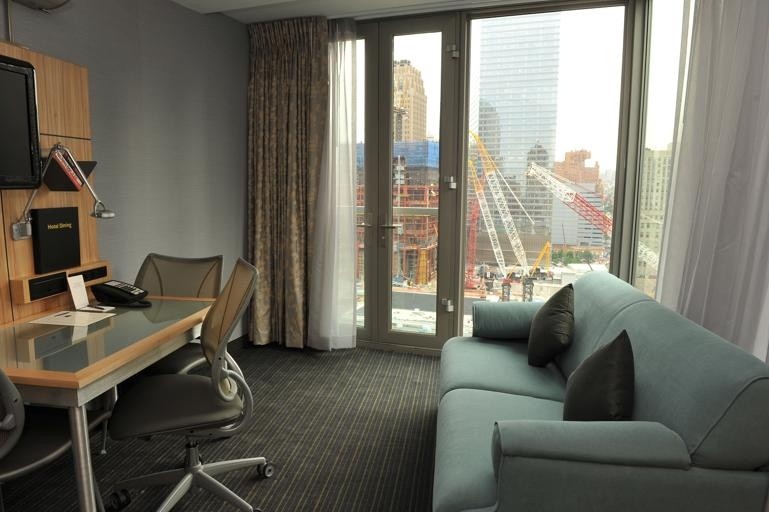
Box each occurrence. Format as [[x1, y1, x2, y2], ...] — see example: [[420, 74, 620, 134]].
[[432, 270, 768, 512]]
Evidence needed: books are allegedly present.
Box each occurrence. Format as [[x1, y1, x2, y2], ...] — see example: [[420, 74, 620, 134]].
[[41, 146, 85, 191]]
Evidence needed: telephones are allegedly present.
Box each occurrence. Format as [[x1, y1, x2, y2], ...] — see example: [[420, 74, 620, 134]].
[[91, 280, 148, 303]]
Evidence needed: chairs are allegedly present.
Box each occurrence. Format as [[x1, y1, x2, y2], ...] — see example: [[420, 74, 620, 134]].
[[0, 252, 275, 510]]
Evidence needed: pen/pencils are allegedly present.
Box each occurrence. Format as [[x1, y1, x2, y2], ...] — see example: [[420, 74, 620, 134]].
[[88, 305, 104, 310]]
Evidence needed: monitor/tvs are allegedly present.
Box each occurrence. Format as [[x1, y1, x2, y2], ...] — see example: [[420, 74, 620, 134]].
[[1, 53, 43, 190]]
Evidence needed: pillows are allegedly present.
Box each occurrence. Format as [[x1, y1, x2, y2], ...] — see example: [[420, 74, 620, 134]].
[[562, 329, 633, 420], [524, 284, 575, 367]]
